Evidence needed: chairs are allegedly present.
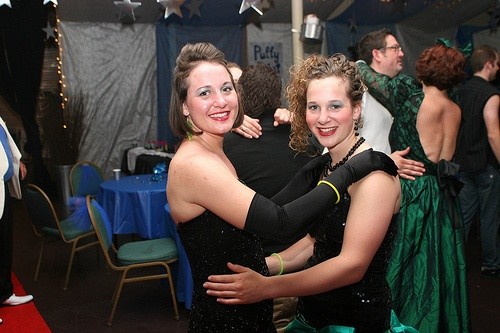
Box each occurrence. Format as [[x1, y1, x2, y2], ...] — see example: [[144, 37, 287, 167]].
[[23, 184, 109, 291], [86, 195, 180, 326], [69, 162, 105, 199]]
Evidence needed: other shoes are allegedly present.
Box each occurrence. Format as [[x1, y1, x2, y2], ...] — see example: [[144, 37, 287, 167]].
[[481, 267, 500, 277], [2, 294, 33, 305]]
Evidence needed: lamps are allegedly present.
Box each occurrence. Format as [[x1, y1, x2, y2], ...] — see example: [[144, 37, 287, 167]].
[[300, 13, 322, 45]]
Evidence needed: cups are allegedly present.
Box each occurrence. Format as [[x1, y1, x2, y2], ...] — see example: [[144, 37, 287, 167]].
[[112, 168, 122, 180]]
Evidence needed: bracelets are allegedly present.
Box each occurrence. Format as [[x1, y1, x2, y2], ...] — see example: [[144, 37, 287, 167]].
[[271, 253, 284, 276], [317, 180, 340, 205]]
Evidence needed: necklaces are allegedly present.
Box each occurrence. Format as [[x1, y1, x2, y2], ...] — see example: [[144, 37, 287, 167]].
[[324, 139, 365, 177], [327, 136, 363, 172]]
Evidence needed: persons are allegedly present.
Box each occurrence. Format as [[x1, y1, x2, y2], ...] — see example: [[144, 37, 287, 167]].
[[166, 27, 500, 332], [0, 99, 33, 325]]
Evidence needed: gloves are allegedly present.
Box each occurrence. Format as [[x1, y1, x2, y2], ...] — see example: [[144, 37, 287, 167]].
[[270, 152, 332, 207], [243, 148, 398, 244]]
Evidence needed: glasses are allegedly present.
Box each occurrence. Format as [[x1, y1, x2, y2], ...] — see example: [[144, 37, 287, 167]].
[[378, 45, 401, 53]]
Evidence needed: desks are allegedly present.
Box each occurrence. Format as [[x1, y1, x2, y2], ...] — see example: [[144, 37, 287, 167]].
[[100, 174, 194, 309], [121, 148, 176, 175]]
